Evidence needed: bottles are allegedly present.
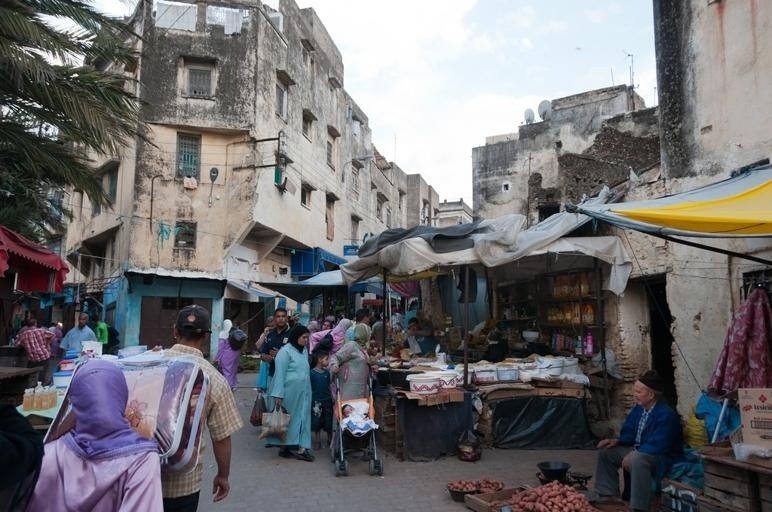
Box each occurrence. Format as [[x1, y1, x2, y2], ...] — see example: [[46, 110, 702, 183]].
[[22, 381, 58, 411], [551, 329, 593, 354], [498, 294, 531, 319], [550, 272, 590, 296], [547, 305, 594, 326]]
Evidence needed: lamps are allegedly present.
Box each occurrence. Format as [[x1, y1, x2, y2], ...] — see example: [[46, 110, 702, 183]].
[[341, 155, 373, 182], [208, 167, 218, 207]]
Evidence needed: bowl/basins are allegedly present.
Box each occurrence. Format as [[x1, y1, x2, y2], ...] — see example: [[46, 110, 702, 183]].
[[536, 460, 571, 479], [521, 329, 539, 343], [537, 472, 571, 485]]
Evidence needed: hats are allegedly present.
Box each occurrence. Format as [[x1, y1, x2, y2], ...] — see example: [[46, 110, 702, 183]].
[[638, 370, 667, 393], [175, 305, 212, 336]]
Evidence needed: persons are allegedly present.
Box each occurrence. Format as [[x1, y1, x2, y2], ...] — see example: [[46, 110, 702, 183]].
[[214, 318, 244, 393], [151, 303, 242, 512], [310, 349, 334, 450], [1, 405, 45, 511], [329, 323, 371, 449], [587, 369, 688, 512], [270, 325, 316, 461], [26, 357, 164, 511], [342, 404, 380, 435], [16, 311, 121, 385], [253, 304, 508, 411]]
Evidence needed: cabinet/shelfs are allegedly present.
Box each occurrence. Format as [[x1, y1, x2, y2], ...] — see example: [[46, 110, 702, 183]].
[[538, 268, 600, 360], [493, 277, 547, 321]]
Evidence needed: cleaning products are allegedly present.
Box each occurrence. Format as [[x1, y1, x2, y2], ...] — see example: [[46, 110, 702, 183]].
[[22, 380, 58, 410]]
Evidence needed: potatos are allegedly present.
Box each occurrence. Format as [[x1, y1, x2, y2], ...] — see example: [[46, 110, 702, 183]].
[[449, 478, 602, 512]]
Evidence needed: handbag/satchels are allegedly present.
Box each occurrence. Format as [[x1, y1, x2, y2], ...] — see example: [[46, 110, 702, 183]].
[[258, 404, 292, 440], [312, 333, 334, 354], [228, 326, 249, 351]]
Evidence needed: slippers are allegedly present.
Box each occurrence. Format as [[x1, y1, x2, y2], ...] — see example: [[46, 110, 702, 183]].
[[294, 450, 316, 462], [277, 448, 294, 457]]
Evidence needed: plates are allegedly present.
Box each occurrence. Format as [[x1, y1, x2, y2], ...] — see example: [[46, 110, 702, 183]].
[[403, 362, 416, 369]]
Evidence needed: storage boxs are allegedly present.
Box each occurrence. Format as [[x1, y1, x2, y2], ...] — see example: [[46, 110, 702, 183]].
[[737, 387, 772, 449]]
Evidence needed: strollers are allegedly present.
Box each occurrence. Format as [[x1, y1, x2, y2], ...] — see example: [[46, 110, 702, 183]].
[[330, 360, 383, 476]]
